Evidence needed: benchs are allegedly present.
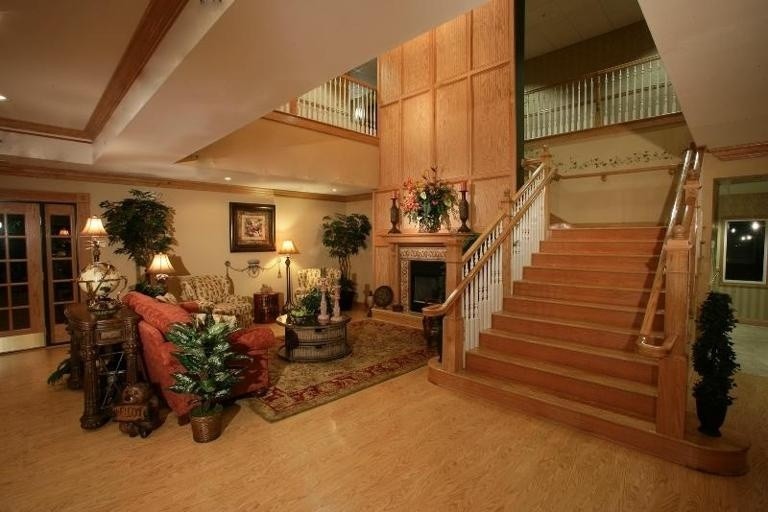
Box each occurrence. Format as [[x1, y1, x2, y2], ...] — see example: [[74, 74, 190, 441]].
[[121, 291, 274, 425]]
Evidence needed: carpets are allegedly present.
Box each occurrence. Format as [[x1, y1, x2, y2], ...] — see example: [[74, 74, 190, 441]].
[[228, 318, 442, 423]]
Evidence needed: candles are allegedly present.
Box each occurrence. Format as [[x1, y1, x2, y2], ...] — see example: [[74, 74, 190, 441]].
[[392, 190, 396, 197], [460, 180, 466, 190]]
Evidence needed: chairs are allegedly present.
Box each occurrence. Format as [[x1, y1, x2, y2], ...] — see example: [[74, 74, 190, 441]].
[[295, 269, 342, 309]]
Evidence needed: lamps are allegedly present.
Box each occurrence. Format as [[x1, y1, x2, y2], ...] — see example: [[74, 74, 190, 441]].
[[147, 252, 175, 284], [79, 216, 107, 262], [278, 240, 300, 312]]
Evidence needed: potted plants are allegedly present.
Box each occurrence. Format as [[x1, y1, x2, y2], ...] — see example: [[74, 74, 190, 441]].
[[691, 291, 741, 437], [165, 322, 237, 442], [322, 213, 372, 311]]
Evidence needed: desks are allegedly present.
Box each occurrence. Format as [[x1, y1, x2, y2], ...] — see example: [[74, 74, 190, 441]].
[[255, 292, 279, 322], [64, 301, 141, 429]]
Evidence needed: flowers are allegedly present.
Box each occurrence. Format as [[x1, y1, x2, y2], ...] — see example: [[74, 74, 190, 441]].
[[400, 166, 458, 230]]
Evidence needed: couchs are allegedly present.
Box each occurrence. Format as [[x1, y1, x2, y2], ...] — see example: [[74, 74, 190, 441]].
[[165, 275, 254, 332]]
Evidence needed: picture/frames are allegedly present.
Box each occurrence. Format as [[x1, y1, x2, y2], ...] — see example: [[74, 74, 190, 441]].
[[229, 202, 276, 253]]
